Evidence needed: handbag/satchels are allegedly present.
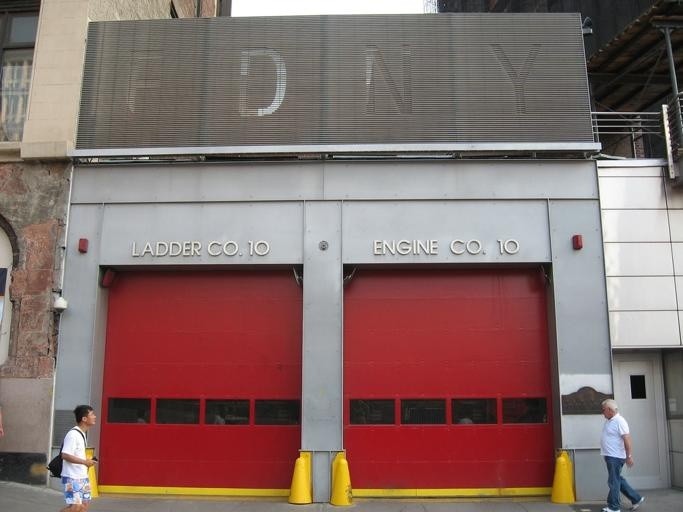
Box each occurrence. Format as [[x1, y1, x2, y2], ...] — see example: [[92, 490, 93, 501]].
[[46, 455, 63, 478]]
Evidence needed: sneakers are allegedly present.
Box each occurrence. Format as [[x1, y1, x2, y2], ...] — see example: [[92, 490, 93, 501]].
[[602, 507, 620, 512], [632, 497, 645, 511]]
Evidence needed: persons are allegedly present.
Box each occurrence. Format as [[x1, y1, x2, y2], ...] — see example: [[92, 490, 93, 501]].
[[45, 404, 98, 511], [598, 399, 646, 511]]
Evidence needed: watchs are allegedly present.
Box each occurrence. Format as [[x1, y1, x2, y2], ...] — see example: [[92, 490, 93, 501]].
[[626, 453, 633, 458]]
